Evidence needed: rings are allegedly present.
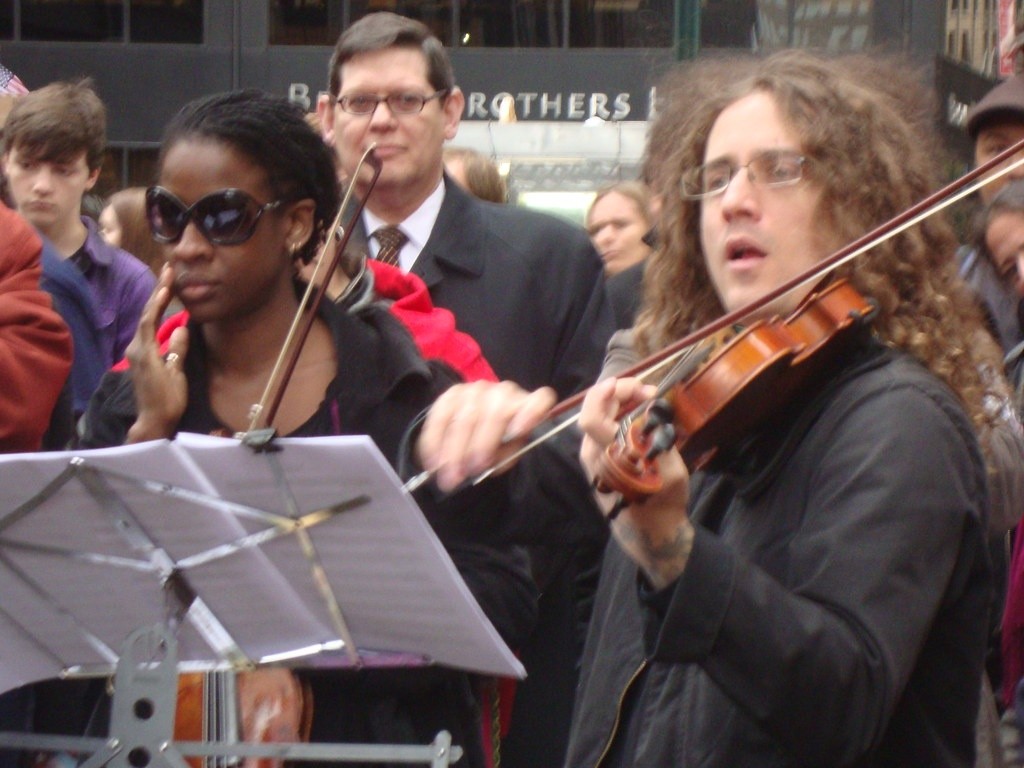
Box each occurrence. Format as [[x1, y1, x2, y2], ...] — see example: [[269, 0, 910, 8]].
[[164, 353, 182, 365]]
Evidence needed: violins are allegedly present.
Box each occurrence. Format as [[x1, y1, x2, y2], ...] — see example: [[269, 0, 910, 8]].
[[175, 665, 312, 768], [592, 275, 881, 503]]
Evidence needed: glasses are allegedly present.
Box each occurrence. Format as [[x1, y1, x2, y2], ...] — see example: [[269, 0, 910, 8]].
[[679, 149, 816, 198], [145, 185, 291, 246], [331, 86, 449, 116]]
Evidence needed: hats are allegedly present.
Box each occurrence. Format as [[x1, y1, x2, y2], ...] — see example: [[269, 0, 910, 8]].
[[966, 74, 1024, 143]]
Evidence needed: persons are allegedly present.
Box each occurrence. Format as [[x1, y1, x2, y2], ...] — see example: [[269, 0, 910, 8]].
[[0, 11, 166, 767], [396, 45, 999, 767], [77, 93, 537, 767], [949, 72, 1024, 768], [288, 11, 655, 768]]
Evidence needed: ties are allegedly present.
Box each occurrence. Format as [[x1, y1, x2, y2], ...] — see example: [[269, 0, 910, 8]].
[[372, 226, 409, 268]]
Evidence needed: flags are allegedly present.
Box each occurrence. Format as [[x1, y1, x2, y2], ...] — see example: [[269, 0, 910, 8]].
[[0, 64, 29, 97]]
